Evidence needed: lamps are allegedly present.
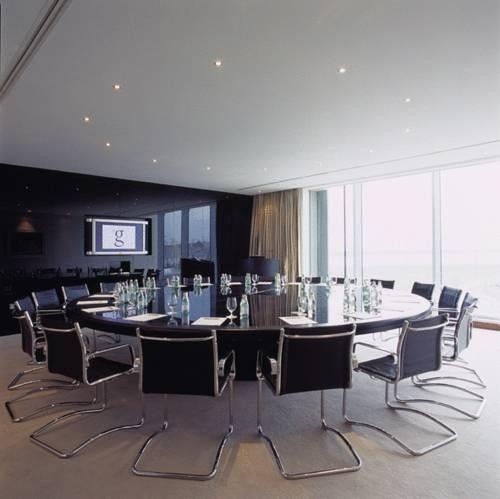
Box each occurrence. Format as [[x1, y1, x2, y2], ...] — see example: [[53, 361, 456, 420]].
[[13, 214, 36, 233]]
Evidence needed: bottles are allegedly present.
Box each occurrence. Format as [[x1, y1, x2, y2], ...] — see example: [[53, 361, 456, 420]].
[[112, 272, 383, 330]]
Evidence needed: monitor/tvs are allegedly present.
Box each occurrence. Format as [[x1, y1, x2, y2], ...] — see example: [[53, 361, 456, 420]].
[[84, 214, 152, 256]]
